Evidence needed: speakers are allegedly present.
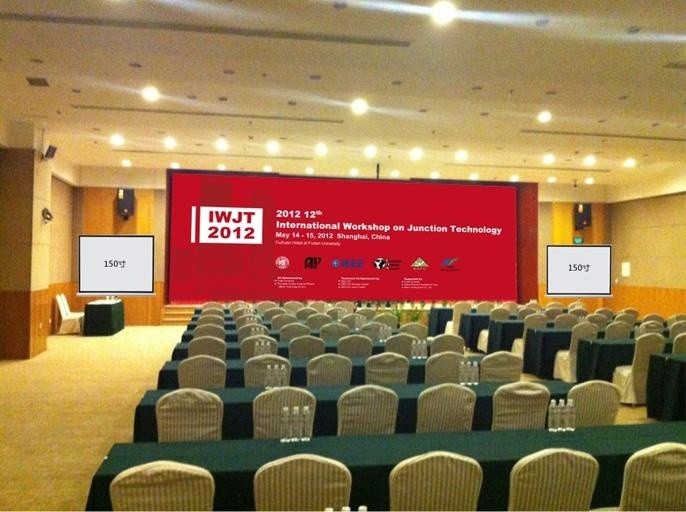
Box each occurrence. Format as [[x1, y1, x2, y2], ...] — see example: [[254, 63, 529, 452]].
[[118, 188, 134, 216], [575, 203, 591, 229]]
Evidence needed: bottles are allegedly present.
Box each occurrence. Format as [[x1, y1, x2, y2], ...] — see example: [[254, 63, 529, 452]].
[[566, 399, 576, 432], [558, 399, 566, 432], [324, 507, 333, 511], [239, 299, 289, 389], [548, 398, 558, 432], [341, 506, 351, 511], [301, 406, 311, 441], [321, 300, 480, 386], [358, 505, 367, 511], [290, 405, 300, 442], [279, 406, 290, 443]]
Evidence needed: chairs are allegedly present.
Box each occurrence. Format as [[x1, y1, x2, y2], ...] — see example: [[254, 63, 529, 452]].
[[55, 293, 83, 335]]
